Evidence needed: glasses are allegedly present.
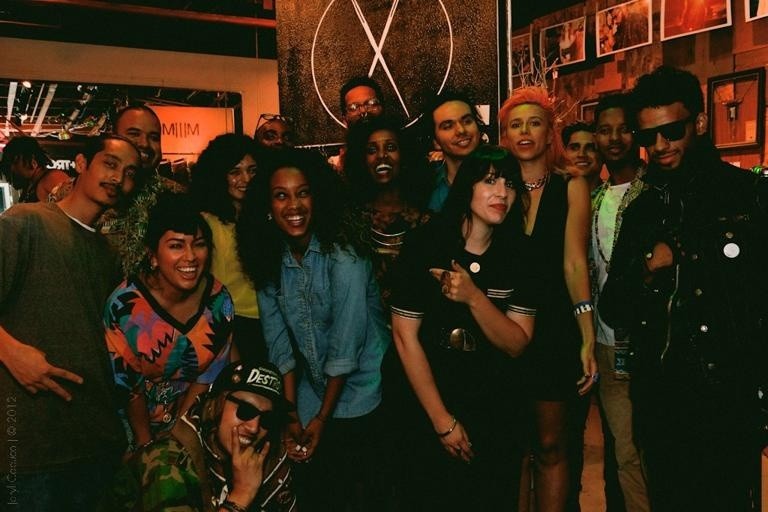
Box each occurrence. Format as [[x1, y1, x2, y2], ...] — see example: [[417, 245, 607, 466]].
[[225, 394, 278, 429], [255, 113, 296, 130], [632, 117, 691, 147], [346, 98, 382, 114]]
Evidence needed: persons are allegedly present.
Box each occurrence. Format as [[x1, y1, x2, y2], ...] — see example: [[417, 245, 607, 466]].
[[1, 66, 768, 512]]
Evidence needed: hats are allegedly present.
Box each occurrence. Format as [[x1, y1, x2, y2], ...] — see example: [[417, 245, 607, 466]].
[[211, 359, 296, 412]]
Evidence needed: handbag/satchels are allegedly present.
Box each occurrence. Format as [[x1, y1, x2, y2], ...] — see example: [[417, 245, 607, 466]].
[[253, 448, 298, 511]]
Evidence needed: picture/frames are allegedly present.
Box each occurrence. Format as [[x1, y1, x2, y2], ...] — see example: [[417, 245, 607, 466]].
[[576, 99, 600, 123], [705, 66, 766, 152]]
